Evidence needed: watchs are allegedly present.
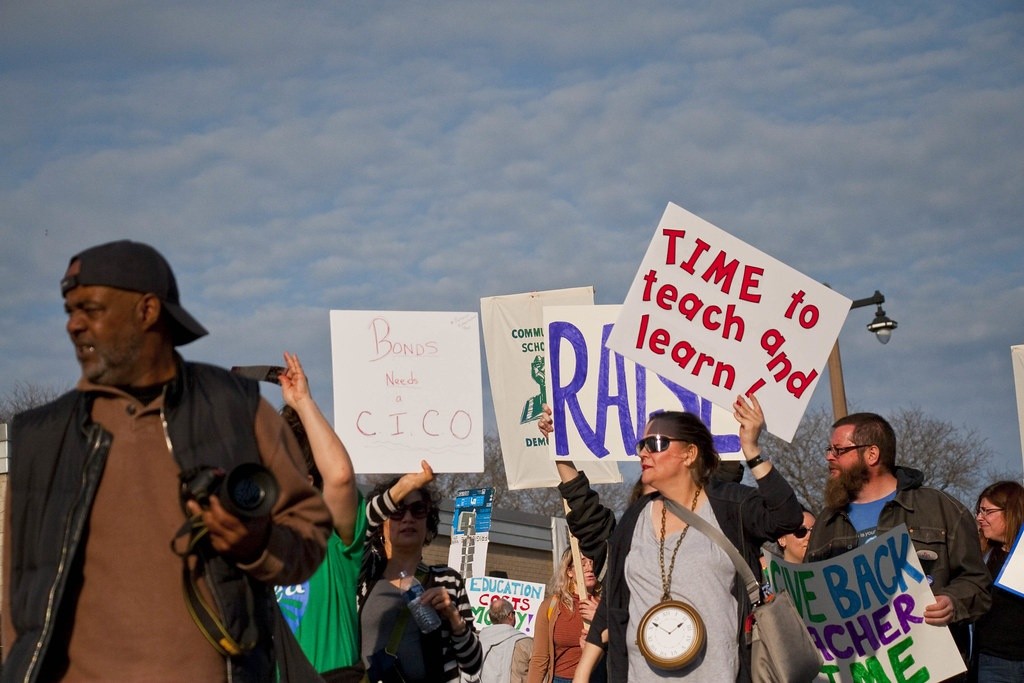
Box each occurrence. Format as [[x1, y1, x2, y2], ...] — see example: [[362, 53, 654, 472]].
[[747, 451, 769, 468]]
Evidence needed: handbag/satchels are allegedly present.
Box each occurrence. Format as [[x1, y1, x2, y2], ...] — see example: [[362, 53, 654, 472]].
[[663, 497, 824, 683]]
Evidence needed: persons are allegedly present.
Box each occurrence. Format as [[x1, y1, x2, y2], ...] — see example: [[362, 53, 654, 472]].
[[355, 460, 483, 683], [460, 599, 533, 683], [0, 240, 334, 683], [268, 351, 368, 683], [528, 394, 1024, 683]]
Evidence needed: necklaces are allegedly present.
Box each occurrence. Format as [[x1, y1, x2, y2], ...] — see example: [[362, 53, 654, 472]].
[[636, 487, 706, 670]]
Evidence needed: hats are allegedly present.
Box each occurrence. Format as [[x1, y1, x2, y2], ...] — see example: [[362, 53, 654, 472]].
[[60, 239, 210, 346]]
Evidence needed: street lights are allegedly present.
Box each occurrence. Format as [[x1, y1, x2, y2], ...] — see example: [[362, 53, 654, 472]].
[[818, 284, 899, 423]]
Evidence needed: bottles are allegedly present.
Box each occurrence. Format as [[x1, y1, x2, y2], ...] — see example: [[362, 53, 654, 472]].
[[397, 570, 442, 634]]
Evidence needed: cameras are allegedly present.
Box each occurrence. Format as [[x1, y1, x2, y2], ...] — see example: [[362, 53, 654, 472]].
[[176, 461, 280, 561]]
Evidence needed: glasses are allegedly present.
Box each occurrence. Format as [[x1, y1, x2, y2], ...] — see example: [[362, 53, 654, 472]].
[[636, 436, 689, 455], [792, 525, 813, 538], [390, 500, 432, 521], [979, 507, 1006, 516], [823, 445, 872, 457]]
